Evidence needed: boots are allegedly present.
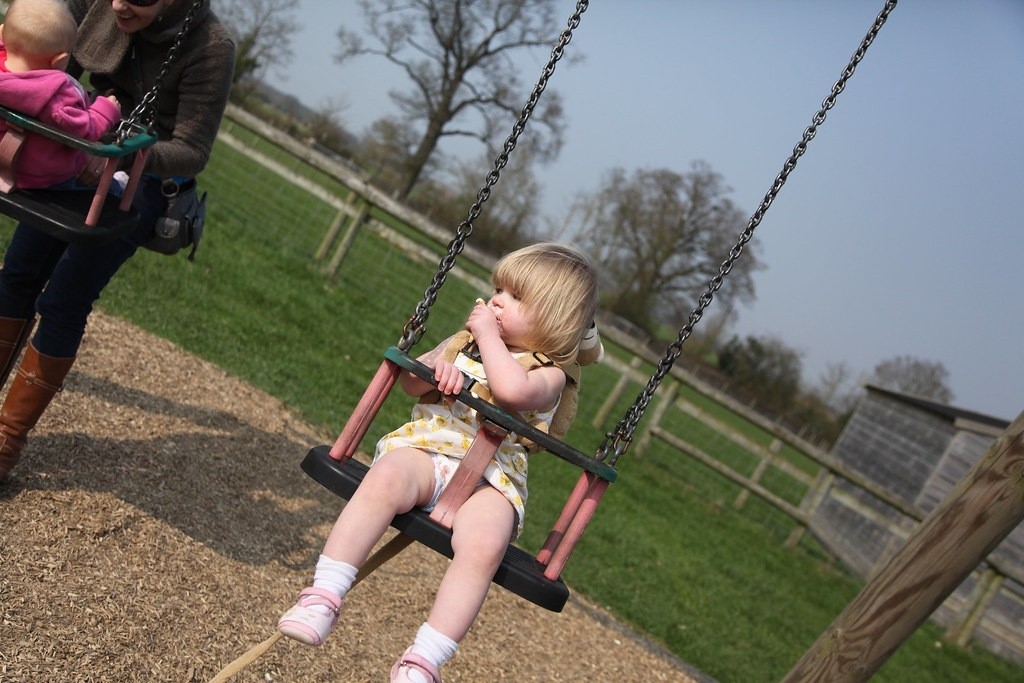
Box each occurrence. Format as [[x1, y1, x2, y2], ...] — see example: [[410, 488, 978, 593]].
[[0, 343, 76, 479], [0, 316, 38, 392]]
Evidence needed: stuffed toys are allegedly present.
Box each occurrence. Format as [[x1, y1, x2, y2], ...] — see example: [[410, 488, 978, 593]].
[[520, 320, 607, 454]]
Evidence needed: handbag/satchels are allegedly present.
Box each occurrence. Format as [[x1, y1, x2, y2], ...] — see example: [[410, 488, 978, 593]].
[[145, 181, 207, 262]]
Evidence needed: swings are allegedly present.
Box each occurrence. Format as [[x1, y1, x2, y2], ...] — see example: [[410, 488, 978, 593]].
[[2, 0, 207, 249], [300, 0, 904, 612]]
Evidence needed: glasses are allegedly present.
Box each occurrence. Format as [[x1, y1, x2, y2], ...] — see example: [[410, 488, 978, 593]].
[[128, 0, 159, 7]]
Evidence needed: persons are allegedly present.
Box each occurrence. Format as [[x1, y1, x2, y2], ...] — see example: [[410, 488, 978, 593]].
[[277, 243, 597, 683], [0, 0, 242, 487]]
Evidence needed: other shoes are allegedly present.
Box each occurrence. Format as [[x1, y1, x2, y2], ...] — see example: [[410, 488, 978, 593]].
[[278, 586, 342, 647], [391, 646, 442, 683]]
